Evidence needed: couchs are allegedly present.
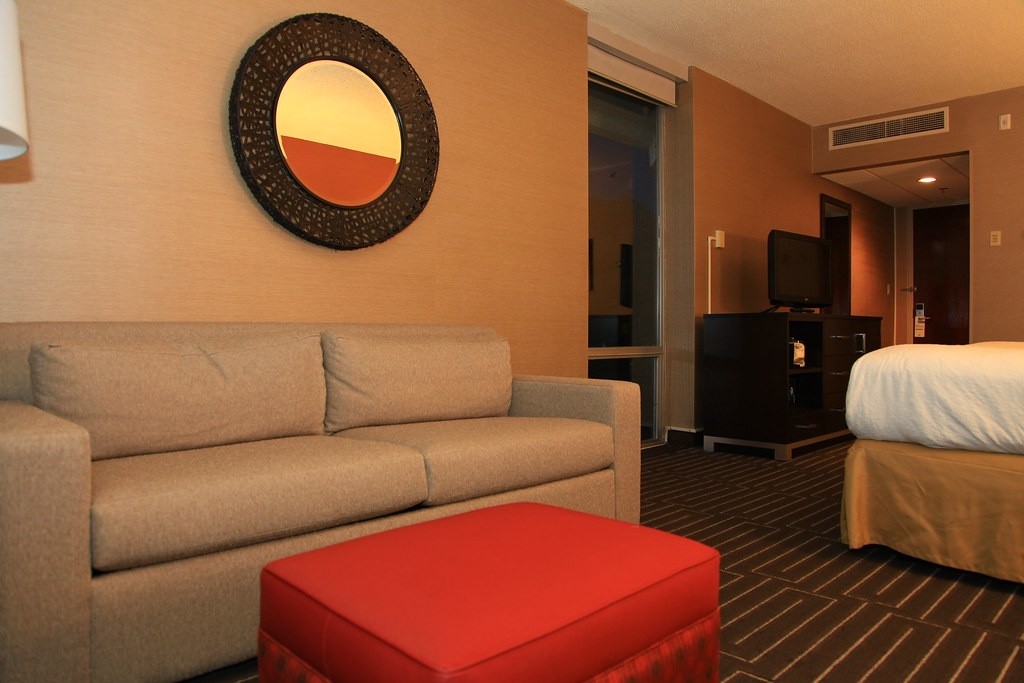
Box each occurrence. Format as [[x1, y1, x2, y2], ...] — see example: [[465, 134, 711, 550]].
[[1, 320, 642, 683]]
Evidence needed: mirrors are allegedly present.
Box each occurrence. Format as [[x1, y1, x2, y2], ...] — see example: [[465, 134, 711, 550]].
[[819, 192, 851, 315], [228, 10, 440, 251]]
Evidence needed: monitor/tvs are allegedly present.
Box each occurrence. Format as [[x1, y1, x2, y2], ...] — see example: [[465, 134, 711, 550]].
[[766, 230, 833, 313]]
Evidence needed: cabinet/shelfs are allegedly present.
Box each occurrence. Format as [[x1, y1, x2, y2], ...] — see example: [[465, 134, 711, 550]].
[[702, 312, 882, 443]]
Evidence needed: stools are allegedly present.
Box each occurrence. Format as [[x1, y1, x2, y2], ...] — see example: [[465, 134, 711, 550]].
[[261, 501, 723, 683]]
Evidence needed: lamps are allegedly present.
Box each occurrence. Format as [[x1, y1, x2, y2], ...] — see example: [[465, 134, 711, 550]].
[[0, 0, 30, 161]]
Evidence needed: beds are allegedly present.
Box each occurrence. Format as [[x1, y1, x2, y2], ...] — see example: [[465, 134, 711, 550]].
[[839, 341, 1023, 584]]
